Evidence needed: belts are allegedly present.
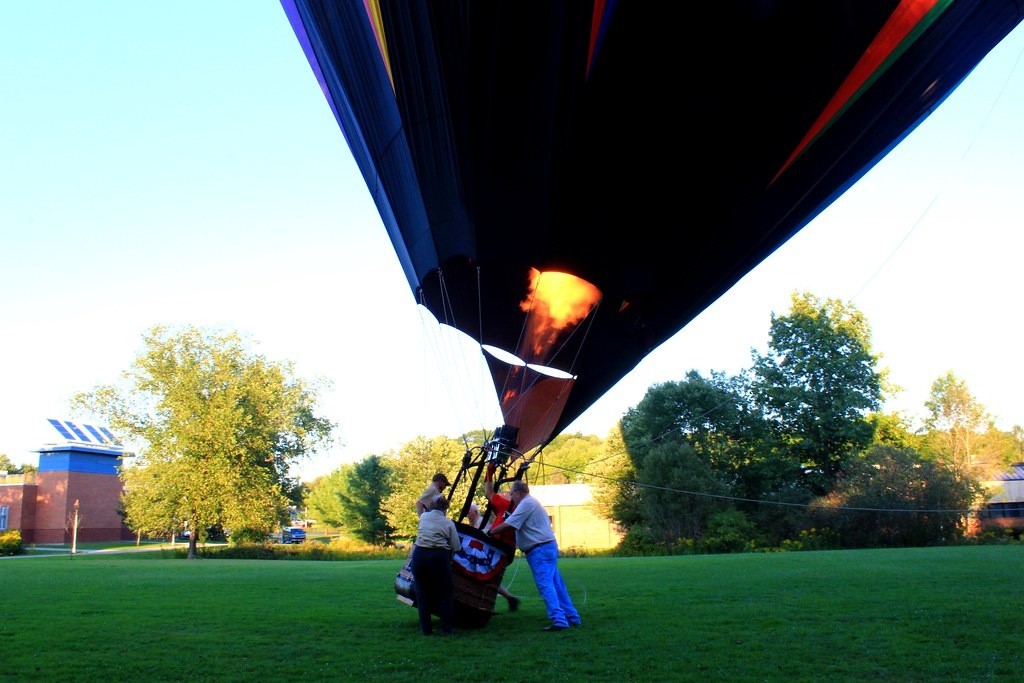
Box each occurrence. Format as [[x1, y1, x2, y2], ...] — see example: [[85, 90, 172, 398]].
[[525, 540, 556, 554]]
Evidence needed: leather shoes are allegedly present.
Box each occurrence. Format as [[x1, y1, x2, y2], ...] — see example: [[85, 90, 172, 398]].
[[544, 625, 569, 631]]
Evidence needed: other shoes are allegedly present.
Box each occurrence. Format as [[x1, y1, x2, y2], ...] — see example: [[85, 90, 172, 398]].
[[425, 630, 455, 635]]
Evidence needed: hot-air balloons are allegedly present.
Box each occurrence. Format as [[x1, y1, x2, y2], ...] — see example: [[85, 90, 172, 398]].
[[276, 3, 1023, 638]]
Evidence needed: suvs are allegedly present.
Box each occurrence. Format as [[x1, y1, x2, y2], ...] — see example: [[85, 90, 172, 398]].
[[282, 527, 307, 544]]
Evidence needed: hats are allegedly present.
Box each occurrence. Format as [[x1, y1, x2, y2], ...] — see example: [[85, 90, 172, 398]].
[[433, 473, 451, 486]]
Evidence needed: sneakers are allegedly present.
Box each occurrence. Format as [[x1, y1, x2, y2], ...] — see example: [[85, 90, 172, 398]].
[[509, 597, 521, 611]]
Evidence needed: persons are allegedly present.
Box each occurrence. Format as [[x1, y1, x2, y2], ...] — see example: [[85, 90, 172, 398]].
[[479, 462, 519, 612], [465, 503, 493, 535], [412, 492, 465, 635], [488, 480, 582, 631], [415, 472, 451, 517]]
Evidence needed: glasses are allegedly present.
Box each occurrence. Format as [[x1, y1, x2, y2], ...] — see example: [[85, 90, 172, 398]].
[[511, 489, 521, 495]]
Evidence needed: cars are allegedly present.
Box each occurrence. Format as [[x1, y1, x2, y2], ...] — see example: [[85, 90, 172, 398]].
[[174, 524, 230, 541]]
